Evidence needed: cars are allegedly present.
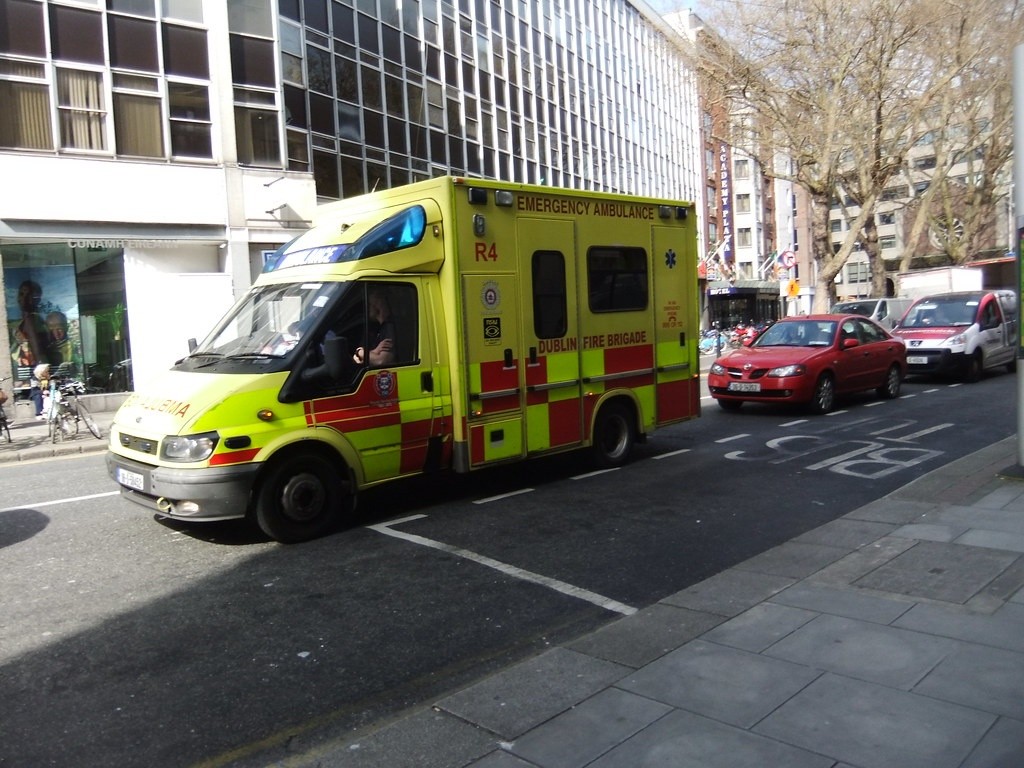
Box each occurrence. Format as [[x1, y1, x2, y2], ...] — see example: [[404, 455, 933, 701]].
[[706, 314, 907, 415], [890, 288, 1019, 382]]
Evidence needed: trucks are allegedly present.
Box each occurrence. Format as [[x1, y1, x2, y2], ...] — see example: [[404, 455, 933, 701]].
[[828, 298, 913, 333]]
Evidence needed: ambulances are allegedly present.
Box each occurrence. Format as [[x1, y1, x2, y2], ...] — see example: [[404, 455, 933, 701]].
[[105, 176, 701, 544]]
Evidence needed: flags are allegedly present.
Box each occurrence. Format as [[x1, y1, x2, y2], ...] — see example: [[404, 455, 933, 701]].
[[711, 239, 737, 287], [771, 250, 790, 271]]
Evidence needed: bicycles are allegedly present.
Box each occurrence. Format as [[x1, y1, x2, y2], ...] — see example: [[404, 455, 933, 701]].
[[0, 376, 12, 444], [48, 377, 102, 444]]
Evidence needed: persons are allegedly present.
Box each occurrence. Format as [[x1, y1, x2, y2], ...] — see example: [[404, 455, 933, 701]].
[[353, 289, 396, 368], [29, 363, 52, 419], [10, 281, 79, 399], [288, 299, 335, 356], [923, 307, 949, 324]]
